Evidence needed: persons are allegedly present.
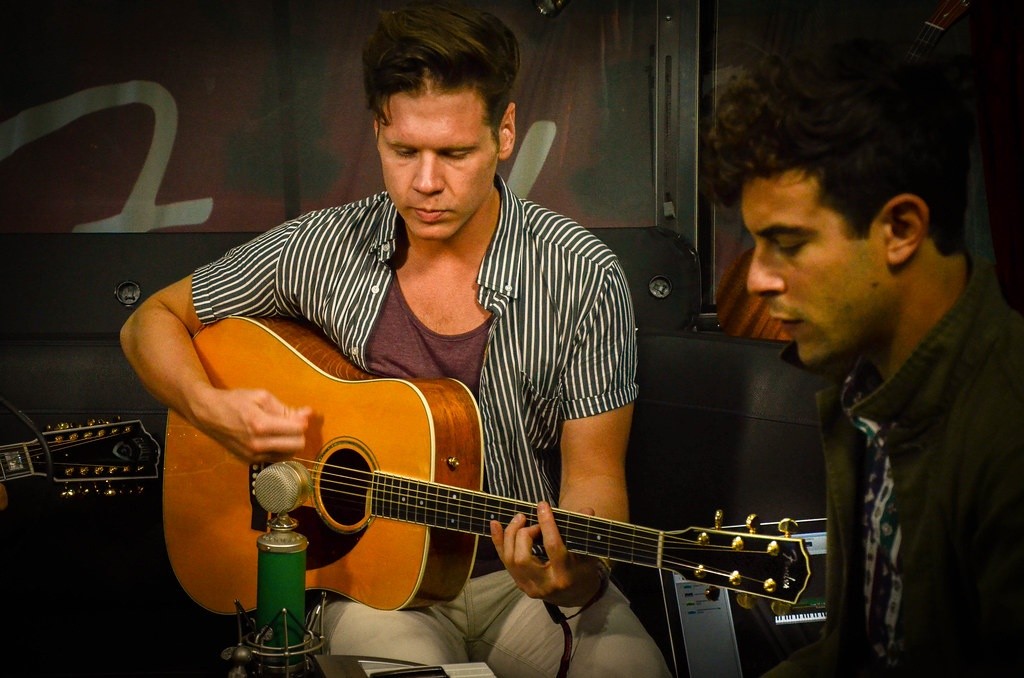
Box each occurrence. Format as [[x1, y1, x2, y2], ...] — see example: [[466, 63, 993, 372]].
[[121, 0, 675, 678], [710, 50, 1024, 678]]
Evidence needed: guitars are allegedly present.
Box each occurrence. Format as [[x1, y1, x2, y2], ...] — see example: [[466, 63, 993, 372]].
[[0, 413, 164, 500], [158, 315, 813, 616]]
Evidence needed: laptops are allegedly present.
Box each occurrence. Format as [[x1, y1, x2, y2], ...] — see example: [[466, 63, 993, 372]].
[[659, 518, 828, 678]]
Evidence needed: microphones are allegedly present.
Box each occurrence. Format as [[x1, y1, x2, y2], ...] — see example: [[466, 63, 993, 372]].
[[255, 461, 312, 678]]
